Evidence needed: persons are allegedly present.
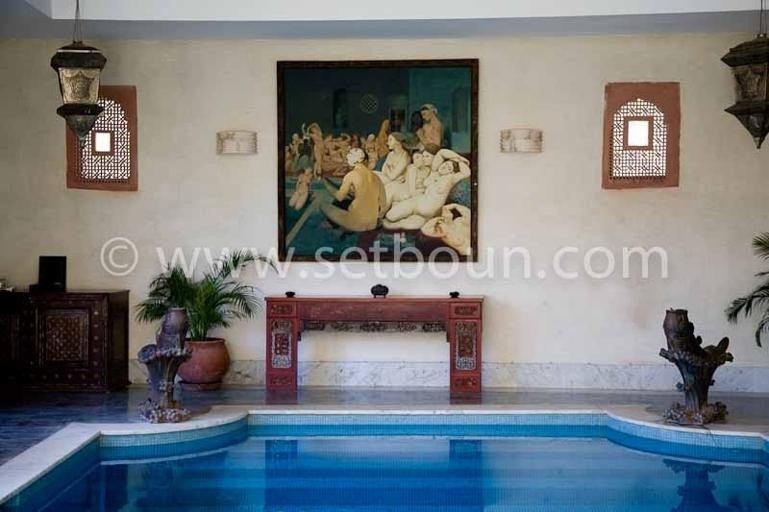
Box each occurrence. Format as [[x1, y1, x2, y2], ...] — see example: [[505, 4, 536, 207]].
[[280, 103, 476, 257]]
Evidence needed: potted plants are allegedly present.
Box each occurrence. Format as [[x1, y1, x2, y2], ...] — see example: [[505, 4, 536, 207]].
[[132, 249, 281, 395]]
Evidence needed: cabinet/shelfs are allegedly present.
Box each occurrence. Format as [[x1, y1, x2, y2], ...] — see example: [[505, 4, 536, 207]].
[[0, 291, 131, 395]]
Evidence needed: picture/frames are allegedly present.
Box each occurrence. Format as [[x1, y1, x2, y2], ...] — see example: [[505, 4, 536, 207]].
[[275, 58, 482, 265]]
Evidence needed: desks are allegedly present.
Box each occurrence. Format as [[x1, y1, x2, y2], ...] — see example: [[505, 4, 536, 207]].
[[263, 294, 486, 406]]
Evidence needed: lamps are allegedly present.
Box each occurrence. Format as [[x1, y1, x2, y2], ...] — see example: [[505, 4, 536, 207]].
[[720, 0, 769, 148], [47, 0, 109, 142]]
